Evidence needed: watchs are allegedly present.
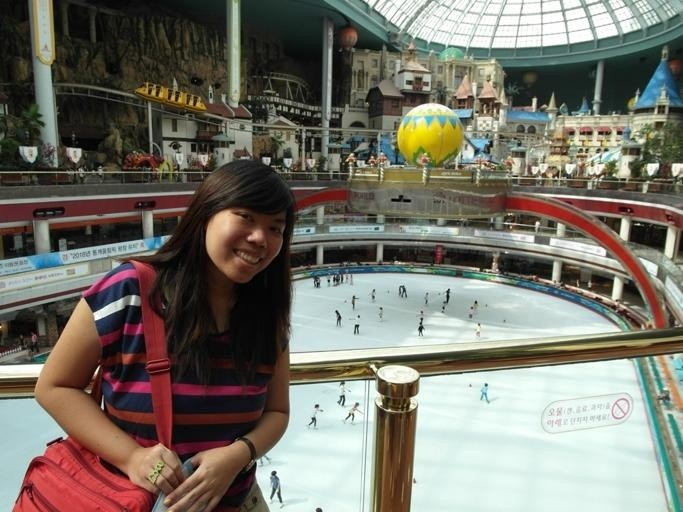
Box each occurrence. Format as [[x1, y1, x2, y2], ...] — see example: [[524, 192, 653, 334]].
[[233, 437, 258, 472]]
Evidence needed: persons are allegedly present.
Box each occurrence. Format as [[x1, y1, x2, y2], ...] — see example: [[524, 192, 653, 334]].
[[315, 508, 322, 512], [19, 335, 26, 350], [31, 331, 40, 353], [443, 287, 452, 305], [479, 382, 491, 404], [440, 304, 446, 313], [307, 260, 431, 428], [468, 300, 479, 320], [260, 455, 270, 466], [34, 160, 298, 512], [476, 323, 482, 336], [587, 280, 592, 290], [269, 471, 283, 505]]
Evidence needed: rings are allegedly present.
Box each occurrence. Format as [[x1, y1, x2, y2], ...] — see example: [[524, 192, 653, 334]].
[[148, 470, 159, 483], [156, 460, 165, 473]]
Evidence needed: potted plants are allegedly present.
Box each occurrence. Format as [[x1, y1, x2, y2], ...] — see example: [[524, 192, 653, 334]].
[[597, 158, 648, 190]]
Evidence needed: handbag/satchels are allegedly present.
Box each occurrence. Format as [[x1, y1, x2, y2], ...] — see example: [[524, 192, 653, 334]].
[[12, 434, 157, 512]]
